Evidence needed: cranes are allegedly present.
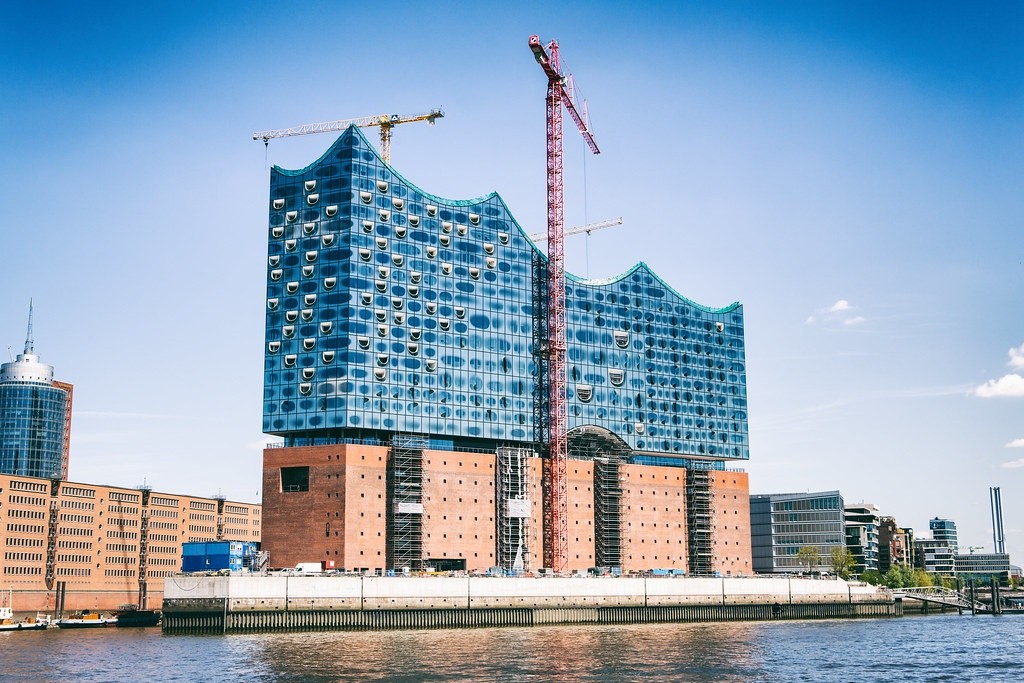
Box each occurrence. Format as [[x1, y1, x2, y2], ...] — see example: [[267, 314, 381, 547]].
[[530, 28, 605, 575], [525, 216, 625, 242], [256, 110, 445, 170]]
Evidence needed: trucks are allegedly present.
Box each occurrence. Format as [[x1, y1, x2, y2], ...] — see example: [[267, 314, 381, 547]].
[[293, 562, 323, 576]]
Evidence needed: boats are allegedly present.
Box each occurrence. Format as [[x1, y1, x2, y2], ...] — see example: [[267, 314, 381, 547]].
[[0, 617, 50, 630], [55, 611, 108, 629], [114, 607, 162, 628]]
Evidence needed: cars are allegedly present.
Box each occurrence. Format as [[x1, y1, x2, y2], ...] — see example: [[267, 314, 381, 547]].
[[278, 568, 295, 577]]
